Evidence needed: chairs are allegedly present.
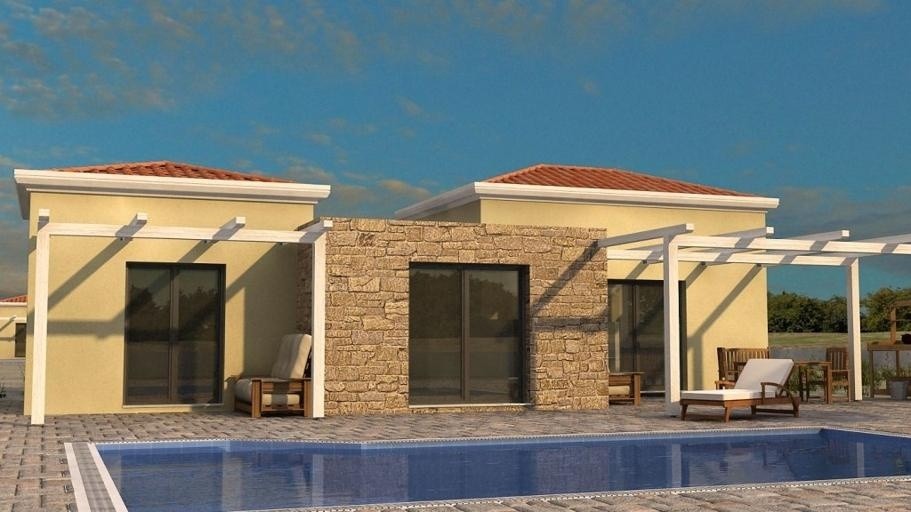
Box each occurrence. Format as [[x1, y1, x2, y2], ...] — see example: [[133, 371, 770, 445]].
[[826, 347, 852, 403], [233, 333, 314, 421], [678, 359, 801, 421]]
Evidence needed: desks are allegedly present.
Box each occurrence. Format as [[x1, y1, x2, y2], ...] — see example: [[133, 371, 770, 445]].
[[735, 361, 832, 405]]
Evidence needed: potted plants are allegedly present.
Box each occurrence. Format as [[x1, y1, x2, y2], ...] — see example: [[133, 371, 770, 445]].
[[861, 359, 881, 399], [885, 367, 910, 401]]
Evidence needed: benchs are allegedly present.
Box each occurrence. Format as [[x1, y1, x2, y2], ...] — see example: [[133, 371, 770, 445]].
[[715, 347, 771, 390]]
